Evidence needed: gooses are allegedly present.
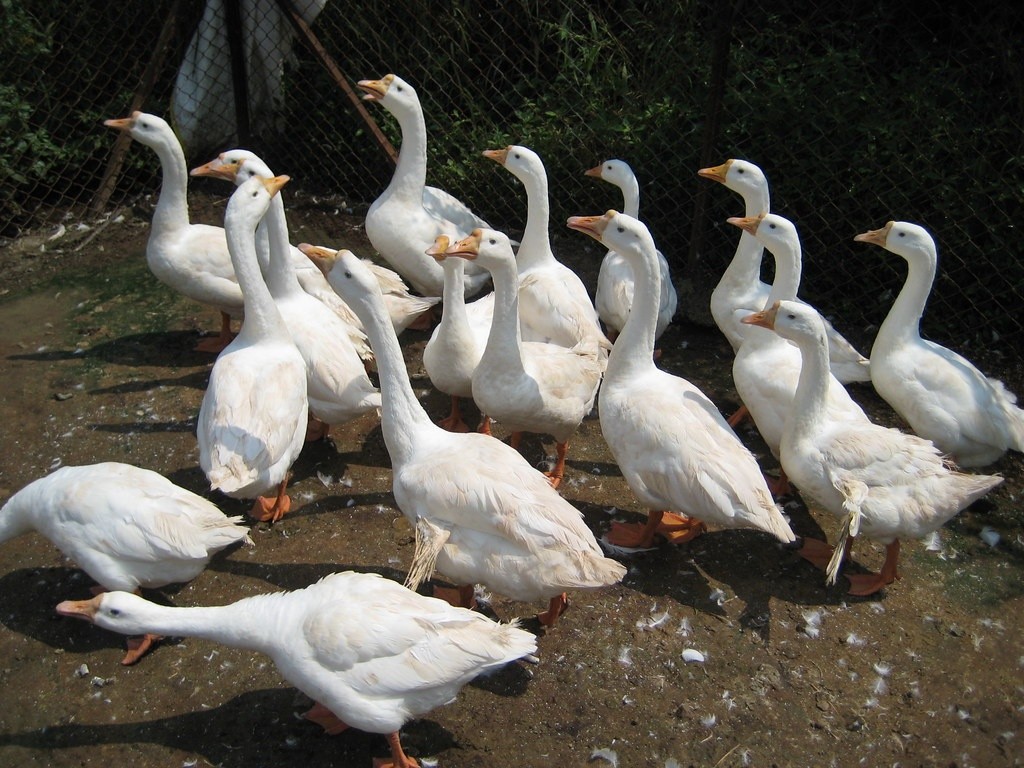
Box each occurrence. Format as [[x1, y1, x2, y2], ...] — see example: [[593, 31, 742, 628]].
[[0, 71, 1024, 768]]
